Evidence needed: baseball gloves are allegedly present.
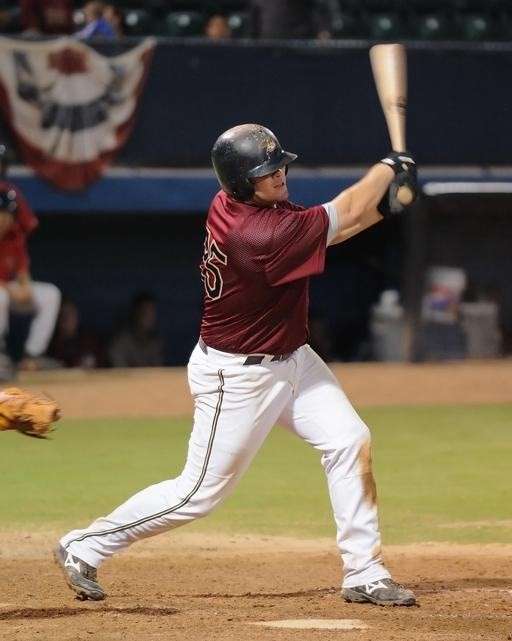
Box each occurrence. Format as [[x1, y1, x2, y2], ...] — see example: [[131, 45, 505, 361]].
[[0, 385, 62, 438]]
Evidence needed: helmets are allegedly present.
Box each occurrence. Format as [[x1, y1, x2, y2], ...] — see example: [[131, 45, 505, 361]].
[[213, 125, 298, 203]]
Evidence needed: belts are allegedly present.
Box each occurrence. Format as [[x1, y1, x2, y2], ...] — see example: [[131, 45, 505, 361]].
[[197, 336, 295, 364]]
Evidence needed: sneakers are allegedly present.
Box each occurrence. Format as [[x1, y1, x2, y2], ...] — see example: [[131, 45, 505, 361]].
[[344, 577, 415, 608], [54, 544, 105, 602]]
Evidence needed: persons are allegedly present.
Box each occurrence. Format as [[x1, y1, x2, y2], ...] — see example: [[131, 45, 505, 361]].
[[0, 142, 70, 378], [243, 0, 343, 45], [104, 290, 170, 370], [46, 294, 108, 368], [205, 13, 235, 41], [50, 124, 424, 607], [0, 0, 130, 43]]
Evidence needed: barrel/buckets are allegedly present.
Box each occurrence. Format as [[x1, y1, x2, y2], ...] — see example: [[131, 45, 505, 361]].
[[369, 264, 500, 363]]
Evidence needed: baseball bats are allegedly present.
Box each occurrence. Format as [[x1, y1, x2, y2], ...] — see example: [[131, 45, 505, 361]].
[[370, 43, 414, 206]]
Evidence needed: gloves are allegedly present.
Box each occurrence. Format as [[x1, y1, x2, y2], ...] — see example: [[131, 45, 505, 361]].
[[376, 151, 418, 219]]
